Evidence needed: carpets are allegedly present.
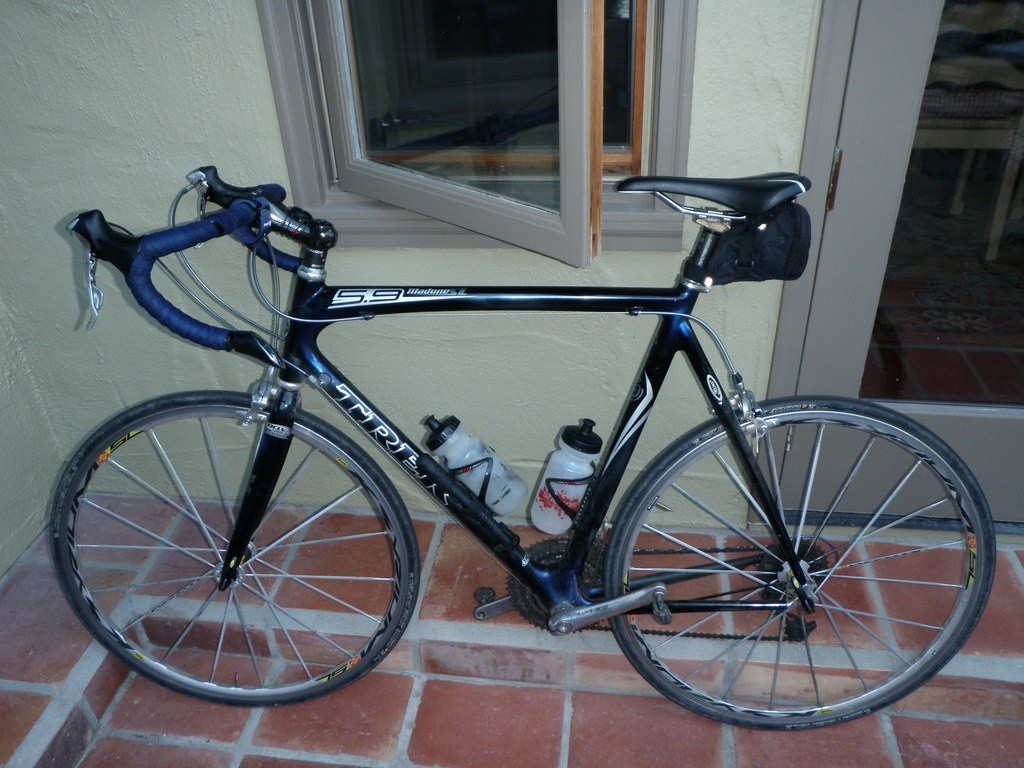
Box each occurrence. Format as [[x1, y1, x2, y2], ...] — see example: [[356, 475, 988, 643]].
[[871, 172, 1024, 346]]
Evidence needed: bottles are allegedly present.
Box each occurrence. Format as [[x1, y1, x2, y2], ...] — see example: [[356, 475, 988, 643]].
[[424, 415, 529, 516], [531, 419, 604, 536]]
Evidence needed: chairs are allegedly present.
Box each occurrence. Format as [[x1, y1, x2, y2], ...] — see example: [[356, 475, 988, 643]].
[[915, 1, 1024, 262]]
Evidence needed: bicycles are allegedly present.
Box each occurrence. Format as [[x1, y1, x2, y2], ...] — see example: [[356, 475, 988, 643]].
[[369, 87, 558, 192], [47, 164, 997, 731]]
